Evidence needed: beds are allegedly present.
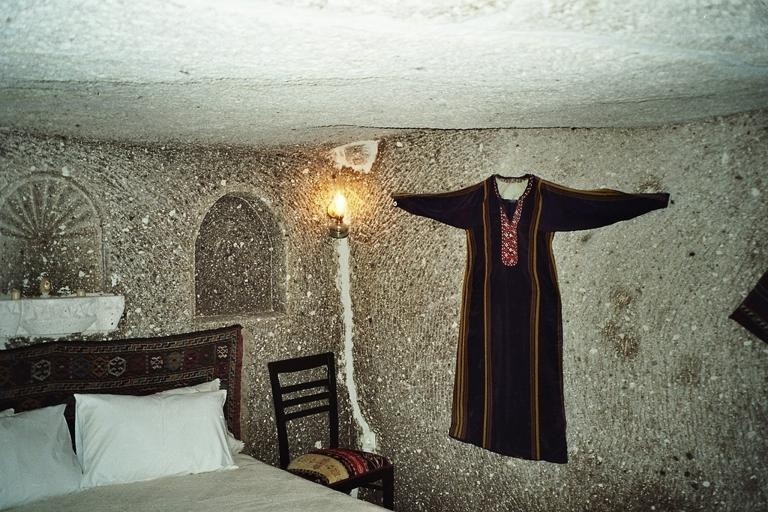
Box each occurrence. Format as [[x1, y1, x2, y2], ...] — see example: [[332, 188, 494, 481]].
[[1, 324, 397, 511]]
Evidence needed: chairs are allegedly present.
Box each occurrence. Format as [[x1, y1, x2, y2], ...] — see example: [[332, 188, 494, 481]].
[[71, 389, 236, 485], [266, 351, 394, 503], [2, 400, 84, 504]]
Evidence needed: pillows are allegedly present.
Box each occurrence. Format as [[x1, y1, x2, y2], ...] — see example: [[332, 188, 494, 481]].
[[152, 374, 245, 451]]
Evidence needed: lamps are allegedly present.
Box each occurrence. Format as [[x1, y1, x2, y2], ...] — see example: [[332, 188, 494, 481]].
[[325, 193, 347, 238]]
